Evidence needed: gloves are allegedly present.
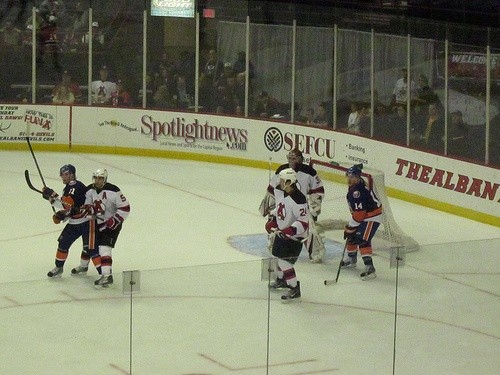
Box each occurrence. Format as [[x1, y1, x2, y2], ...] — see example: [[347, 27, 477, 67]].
[[265, 221, 278, 232], [344, 225, 357, 240], [42, 187, 59, 205], [277, 226, 296, 240], [105, 214, 124, 230], [53, 210, 71, 224], [79, 205, 92, 218]]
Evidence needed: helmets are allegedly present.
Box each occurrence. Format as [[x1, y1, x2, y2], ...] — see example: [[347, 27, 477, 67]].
[[347, 167, 360, 175], [92, 168, 108, 190], [60, 164, 75, 178], [279, 168, 298, 190], [286, 149, 303, 167]]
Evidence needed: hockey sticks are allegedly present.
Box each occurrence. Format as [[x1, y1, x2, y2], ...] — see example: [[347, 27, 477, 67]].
[[324, 234, 351, 286], [271, 227, 313, 243], [26, 122, 57, 215], [25, 170, 105, 222], [269, 157, 272, 184]]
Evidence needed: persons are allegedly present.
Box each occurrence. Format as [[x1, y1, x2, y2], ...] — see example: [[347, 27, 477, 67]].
[[264, 169, 310, 301], [0, 0, 468, 155], [72, 168, 130, 286], [339, 163, 382, 277], [41, 164, 104, 279], [274, 149, 325, 260]]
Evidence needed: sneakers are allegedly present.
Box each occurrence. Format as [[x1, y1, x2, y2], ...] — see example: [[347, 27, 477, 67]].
[[95, 275, 115, 289], [360, 265, 376, 281], [341, 256, 357, 268], [268, 275, 287, 292], [280, 281, 302, 304], [47, 267, 63, 278], [71, 264, 88, 276]]
